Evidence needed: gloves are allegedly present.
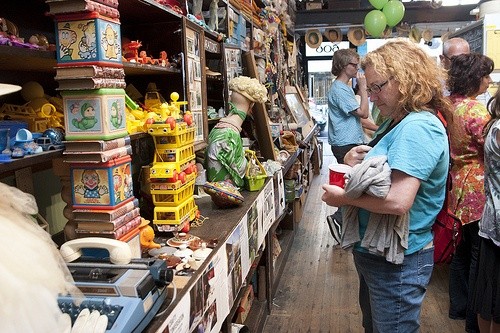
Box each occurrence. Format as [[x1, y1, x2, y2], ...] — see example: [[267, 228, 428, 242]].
[[63, 308, 108, 333]]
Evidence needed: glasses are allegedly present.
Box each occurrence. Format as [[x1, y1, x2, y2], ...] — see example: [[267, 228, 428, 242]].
[[348, 62, 361, 70], [443, 53, 457, 63], [366, 73, 397, 96]]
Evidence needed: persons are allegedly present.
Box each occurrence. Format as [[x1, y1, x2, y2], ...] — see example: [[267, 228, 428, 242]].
[[439, 37, 470, 70], [321, 42, 467, 333], [207, 77, 267, 189], [448, 53, 494, 318], [471, 85, 500, 333], [326, 50, 380, 245]]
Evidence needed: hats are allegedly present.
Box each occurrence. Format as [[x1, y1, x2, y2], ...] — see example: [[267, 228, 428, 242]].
[[396, 25, 408, 35], [382, 27, 393, 38], [324, 26, 343, 42], [441, 32, 453, 41], [422, 29, 434, 42], [305, 29, 323, 48], [409, 27, 421, 43], [347, 28, 365, 45]]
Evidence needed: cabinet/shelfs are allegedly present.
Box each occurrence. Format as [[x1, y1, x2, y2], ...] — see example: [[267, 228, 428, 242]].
[[142, 123, 319, 333], [0, 0, 281, 243]]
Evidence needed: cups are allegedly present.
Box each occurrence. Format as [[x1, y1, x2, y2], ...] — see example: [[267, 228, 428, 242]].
[[328, 164, 353, 189]]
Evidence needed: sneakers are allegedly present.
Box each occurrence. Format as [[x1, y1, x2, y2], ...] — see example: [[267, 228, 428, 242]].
[[326, 214, 344, 244]]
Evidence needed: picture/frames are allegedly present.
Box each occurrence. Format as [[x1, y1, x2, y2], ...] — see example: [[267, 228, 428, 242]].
[[223, 44, 243, 116], [182, 15, 208, 152]]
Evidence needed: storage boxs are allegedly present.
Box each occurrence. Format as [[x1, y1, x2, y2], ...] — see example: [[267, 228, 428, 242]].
[[278, 189, 306, 230]]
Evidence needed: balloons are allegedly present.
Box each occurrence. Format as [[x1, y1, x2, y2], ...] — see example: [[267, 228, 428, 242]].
[[364, 0, 406, 37]]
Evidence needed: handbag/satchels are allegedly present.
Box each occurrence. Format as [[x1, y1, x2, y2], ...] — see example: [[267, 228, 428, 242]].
[[433, 205, 463, 265]]
